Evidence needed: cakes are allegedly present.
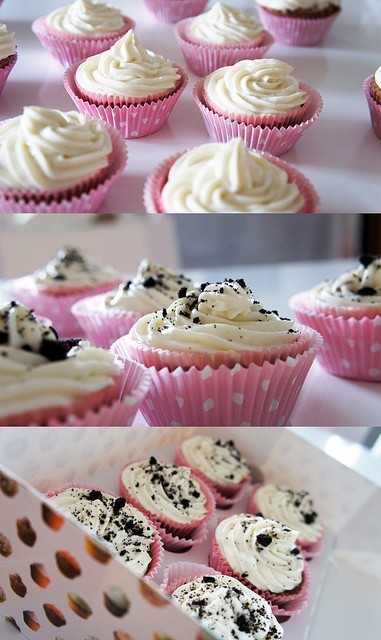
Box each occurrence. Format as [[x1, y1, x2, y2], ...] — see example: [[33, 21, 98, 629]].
[[192, 58, 323, 157], [289, 253, 381, 382], [159, 561, 285, 640], [41, 482, 165, 581], [246, 481, 327, 559], [254, 0, 342, 45], [109, 277, 324, 426], [0, 22, 17, 95], [62, 29, 189, 139], [0, 105, 128, 213], [175, 1, 274, 78], [143, 136, 321, 212], [173, 435, 251, 509], [7, 242, 125, 340], [31, 0, 136, 73], [363, 65, 381, 140], [1, 300, 152, 427], [208, 511, 311, 616], [143, 0, 211, 24], [71, 257, 194, 350], [119, 456, 217, 552]]
